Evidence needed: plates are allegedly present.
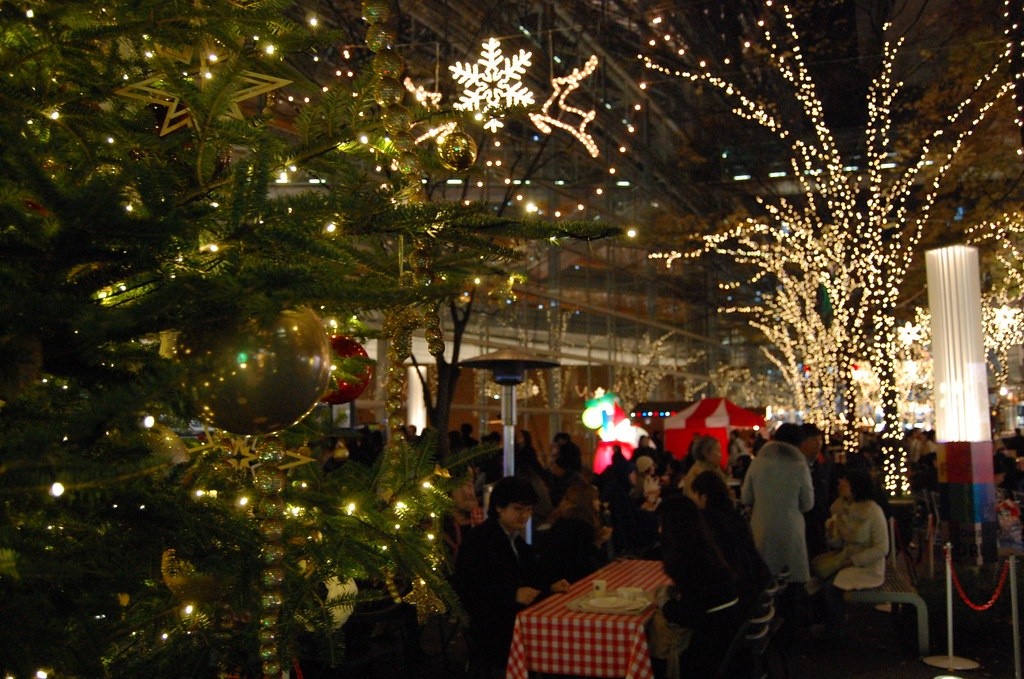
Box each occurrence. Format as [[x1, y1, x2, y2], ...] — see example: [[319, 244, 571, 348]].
[[589, 597, 629, 607]]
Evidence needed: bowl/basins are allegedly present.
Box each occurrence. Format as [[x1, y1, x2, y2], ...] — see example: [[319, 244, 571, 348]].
[[617, 588, 642, 600]]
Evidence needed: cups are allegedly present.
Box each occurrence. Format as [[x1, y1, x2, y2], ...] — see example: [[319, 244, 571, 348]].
[[593, 579, 606, 597]]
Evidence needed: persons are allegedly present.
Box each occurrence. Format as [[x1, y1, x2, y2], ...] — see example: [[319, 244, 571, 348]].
[[307, 424, 1024, 679]]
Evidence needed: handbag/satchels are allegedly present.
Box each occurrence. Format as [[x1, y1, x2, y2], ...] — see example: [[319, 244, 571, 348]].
[[647, 610, 692, 660], [810, 547, 846, 580]]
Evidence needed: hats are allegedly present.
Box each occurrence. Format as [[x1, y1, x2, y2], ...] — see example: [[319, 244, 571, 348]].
[[491, 476, 542, 506], [635, 457, 653, 474]]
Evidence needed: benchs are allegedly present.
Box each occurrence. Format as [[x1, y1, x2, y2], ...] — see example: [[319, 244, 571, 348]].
[[842, 569, 929, 656]]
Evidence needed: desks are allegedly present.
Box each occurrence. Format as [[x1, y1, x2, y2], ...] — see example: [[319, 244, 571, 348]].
[[505, 558, 675, 679]]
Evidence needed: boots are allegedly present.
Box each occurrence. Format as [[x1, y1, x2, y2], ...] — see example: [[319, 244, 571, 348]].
[[810, 614, 848, 639]]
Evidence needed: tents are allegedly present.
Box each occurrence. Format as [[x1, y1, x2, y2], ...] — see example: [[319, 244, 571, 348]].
[[663, 398, 769, 472]]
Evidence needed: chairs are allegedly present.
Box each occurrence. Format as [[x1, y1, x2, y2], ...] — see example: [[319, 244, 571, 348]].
[[678, 563, 793, 679]]
